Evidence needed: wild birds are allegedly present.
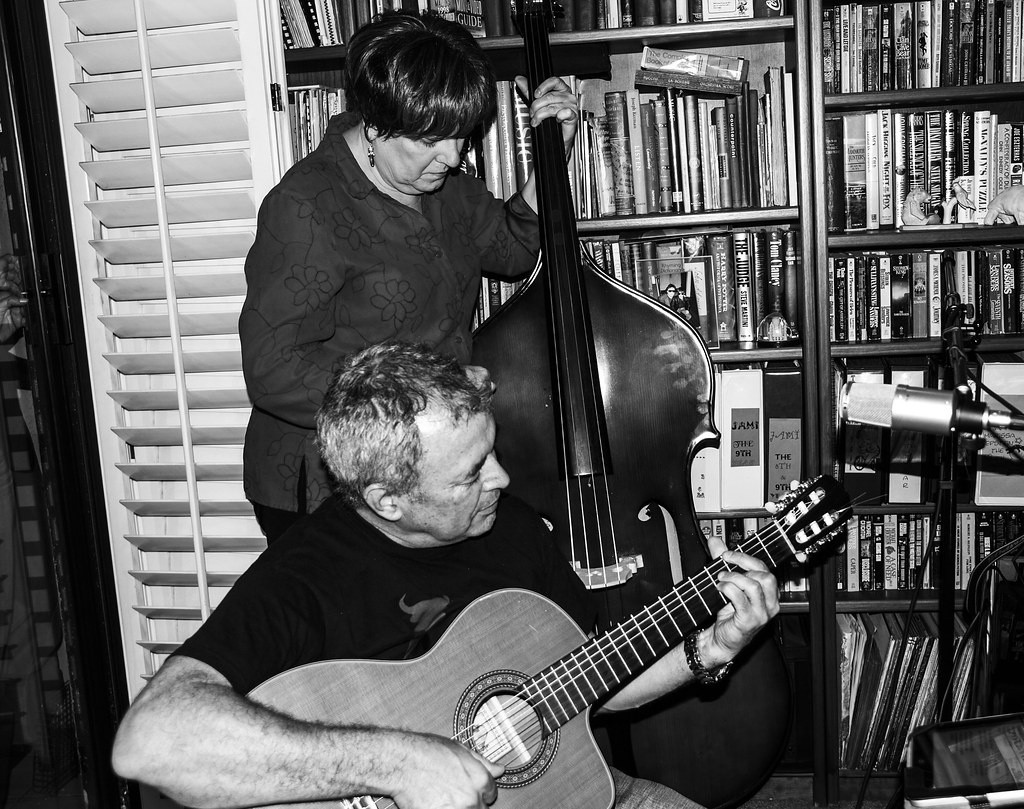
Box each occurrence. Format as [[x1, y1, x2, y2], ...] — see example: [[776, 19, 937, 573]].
[[950, 183, 977, 210]]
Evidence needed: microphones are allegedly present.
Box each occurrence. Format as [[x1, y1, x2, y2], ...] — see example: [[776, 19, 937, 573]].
[[840, 380, 1024, 436]]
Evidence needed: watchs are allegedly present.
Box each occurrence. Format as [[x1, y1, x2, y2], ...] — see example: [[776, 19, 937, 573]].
[[679, 627, 740, 689]]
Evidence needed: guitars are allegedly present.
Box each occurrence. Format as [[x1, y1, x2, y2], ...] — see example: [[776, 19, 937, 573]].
[[245, 476, 890, 809]]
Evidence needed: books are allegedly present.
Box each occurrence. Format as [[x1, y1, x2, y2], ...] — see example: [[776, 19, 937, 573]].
[[473, 224, 1024, 606], [276, 0, 1024, 239], [831, 605, 1024, 772]]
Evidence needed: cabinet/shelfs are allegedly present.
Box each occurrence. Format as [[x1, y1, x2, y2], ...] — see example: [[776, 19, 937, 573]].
[[811, 0, 1024, 809], [267, 0, 821, 809]]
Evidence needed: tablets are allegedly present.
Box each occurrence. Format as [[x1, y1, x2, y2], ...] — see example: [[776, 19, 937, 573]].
[[906, 710, 1024, 809]]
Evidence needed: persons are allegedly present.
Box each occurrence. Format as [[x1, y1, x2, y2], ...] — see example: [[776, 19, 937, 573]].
[[110, 5, 782, 809]]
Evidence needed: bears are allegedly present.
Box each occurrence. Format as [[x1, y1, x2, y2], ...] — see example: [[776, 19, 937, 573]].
[[901, 187, 932, 225]]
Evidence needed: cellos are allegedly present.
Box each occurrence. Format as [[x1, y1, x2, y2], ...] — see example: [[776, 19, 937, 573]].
[[466, 0, 800, 809]]
